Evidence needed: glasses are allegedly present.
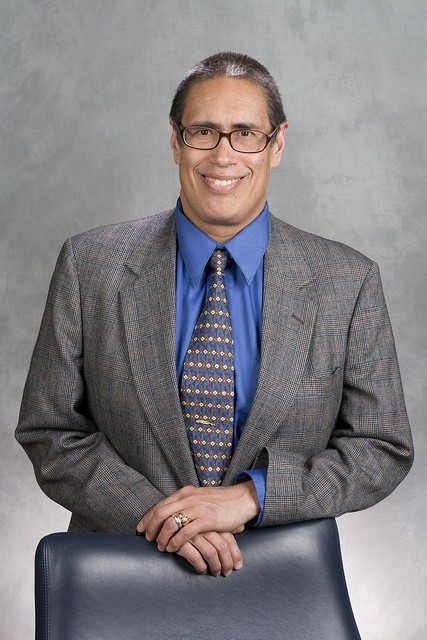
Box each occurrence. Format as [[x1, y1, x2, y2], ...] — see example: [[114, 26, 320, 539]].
[[173, 119, 281, 153]]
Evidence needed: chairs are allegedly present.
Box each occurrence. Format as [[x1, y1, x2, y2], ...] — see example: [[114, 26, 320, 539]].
[[30, 517, 366, 640]]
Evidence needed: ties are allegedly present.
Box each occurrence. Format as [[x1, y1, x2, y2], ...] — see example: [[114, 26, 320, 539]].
[[178, 250, 235, 487]]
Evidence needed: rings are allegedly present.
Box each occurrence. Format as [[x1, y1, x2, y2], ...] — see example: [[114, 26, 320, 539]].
[[171, 514, 182, 528], [177, 512, 189, 526]]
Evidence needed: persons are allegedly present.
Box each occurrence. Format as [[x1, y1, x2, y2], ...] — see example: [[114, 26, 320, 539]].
[[14, 51, 414, 576]]
